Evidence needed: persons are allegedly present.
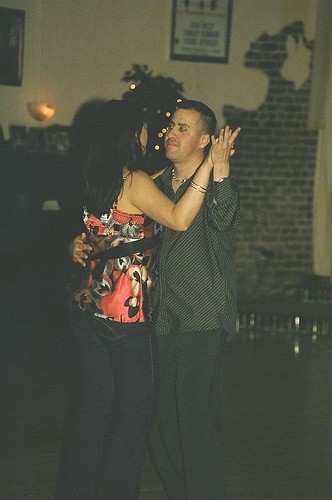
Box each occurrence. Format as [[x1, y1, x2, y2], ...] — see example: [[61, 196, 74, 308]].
[[66, 101, 242, 500], [68, 100, 237, 500]]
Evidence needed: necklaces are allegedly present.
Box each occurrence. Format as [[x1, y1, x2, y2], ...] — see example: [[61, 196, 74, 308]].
[[171, 166, 189, 183]]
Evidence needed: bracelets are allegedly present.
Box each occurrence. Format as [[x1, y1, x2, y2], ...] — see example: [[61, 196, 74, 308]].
[[189, 180, 208, 196]]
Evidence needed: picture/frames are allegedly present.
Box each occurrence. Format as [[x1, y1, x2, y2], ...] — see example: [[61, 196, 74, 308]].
[[168, 0, 235, 64], [0, 7, 26, 87]]
